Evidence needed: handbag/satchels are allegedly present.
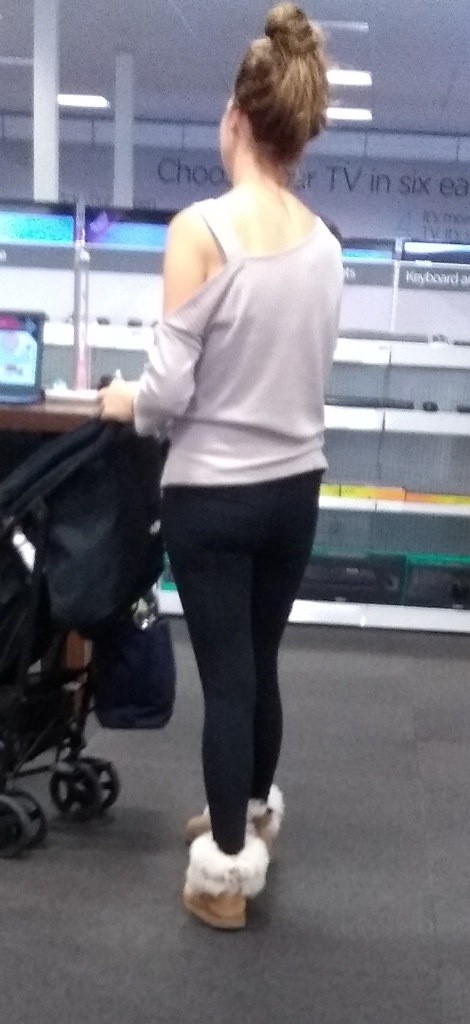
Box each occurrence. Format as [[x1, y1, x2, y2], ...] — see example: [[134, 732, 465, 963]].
[[88, 590, 176, 729]]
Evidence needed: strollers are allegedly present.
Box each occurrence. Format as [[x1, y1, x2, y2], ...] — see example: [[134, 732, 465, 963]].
[[0, 414, 178, 859]]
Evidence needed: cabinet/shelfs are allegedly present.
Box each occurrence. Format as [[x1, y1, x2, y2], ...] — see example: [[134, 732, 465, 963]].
[[0, 197, 470, 634]]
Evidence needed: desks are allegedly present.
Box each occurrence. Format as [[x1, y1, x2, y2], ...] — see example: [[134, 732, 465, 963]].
[[0, 396, 102, 431]]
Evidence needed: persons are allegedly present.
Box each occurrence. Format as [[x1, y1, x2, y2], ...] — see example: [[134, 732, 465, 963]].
[[101, 3, 344, 930]]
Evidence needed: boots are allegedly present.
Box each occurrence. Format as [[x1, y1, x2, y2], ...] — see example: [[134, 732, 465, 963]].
[[182, 831, 270, 929], [186, 784, 284, 851]]
